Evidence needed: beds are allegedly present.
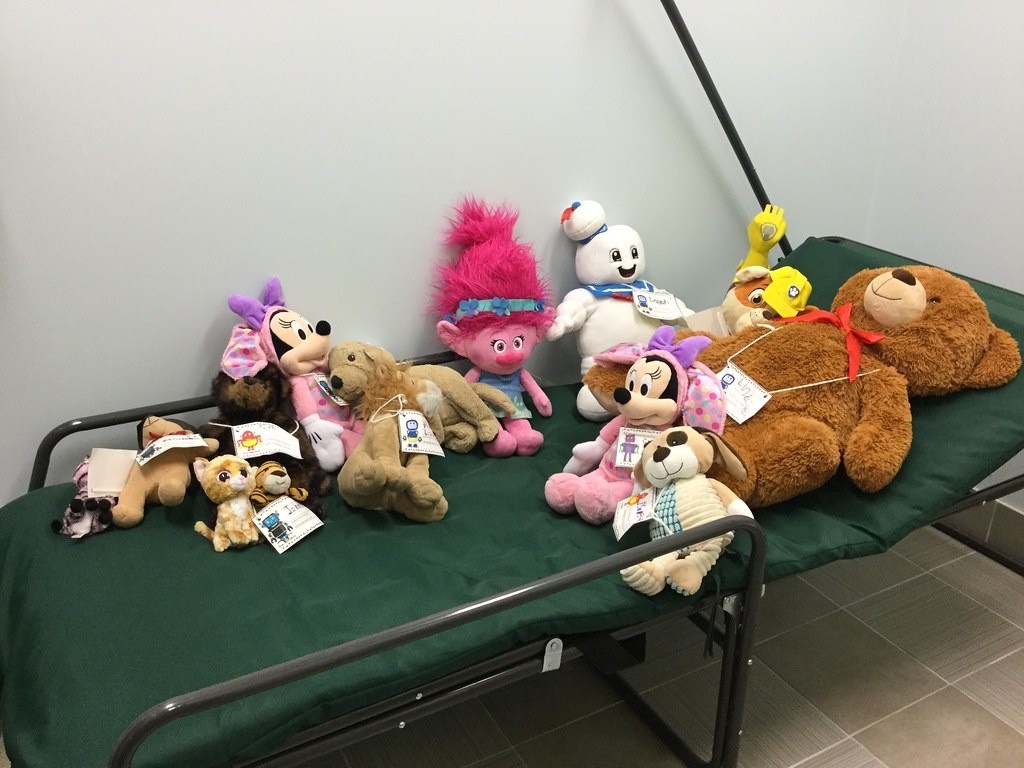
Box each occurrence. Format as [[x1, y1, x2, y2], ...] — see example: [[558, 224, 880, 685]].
[[0, 234, 1024, 768]]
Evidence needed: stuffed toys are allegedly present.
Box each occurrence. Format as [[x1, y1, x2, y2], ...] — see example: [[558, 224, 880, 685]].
[[547, 199, 1022, 597], [50, 275, 517, 553], [426, 192, 556, 457]]
